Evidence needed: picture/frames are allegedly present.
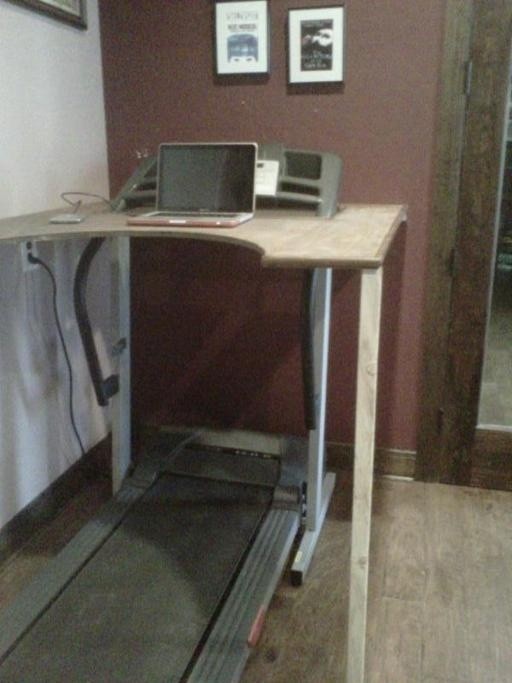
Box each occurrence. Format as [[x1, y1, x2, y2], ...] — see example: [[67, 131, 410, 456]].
[[11, 1, 88, 32], [211, 0, 270, 84], [286, 3, 345, 94]]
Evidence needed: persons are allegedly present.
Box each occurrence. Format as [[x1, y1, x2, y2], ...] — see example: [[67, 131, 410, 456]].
[[227, 35, 258, 66], [304, 20, 332, 71]]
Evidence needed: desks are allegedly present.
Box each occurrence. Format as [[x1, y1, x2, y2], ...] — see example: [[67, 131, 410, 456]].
[[0, 201, 408, 589]]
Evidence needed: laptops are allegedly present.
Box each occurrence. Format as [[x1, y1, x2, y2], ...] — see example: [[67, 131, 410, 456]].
[[124, 141, 259, 229]]
